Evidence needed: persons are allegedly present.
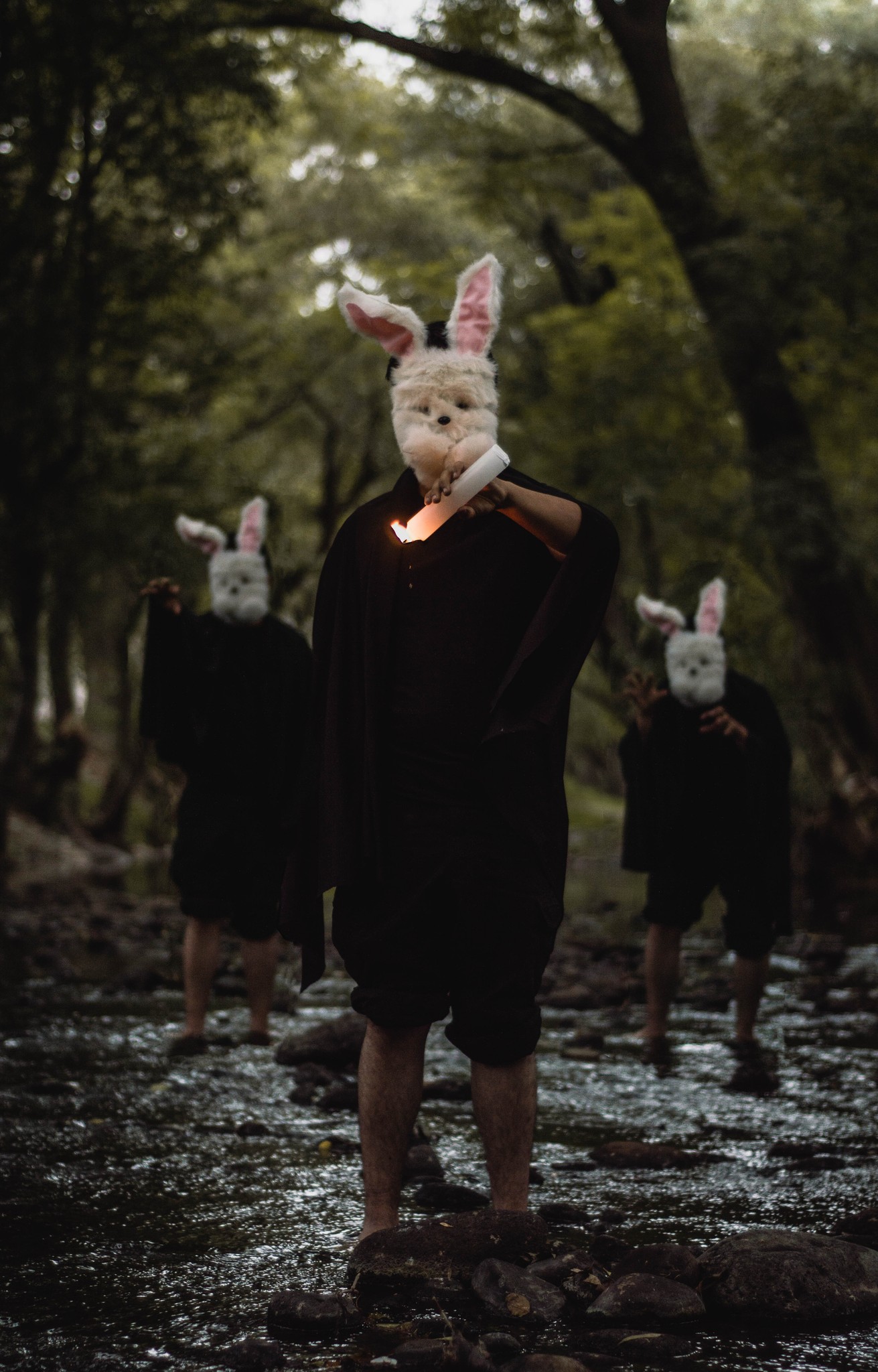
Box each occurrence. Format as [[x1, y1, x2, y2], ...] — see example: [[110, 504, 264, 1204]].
[[615, 574, 793, 1058], [138, 481, 313, 1060], [291, 254, 623, 1243]]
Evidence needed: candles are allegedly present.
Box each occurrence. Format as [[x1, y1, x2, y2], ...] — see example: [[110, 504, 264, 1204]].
[[402, 445, 510, 542]]
